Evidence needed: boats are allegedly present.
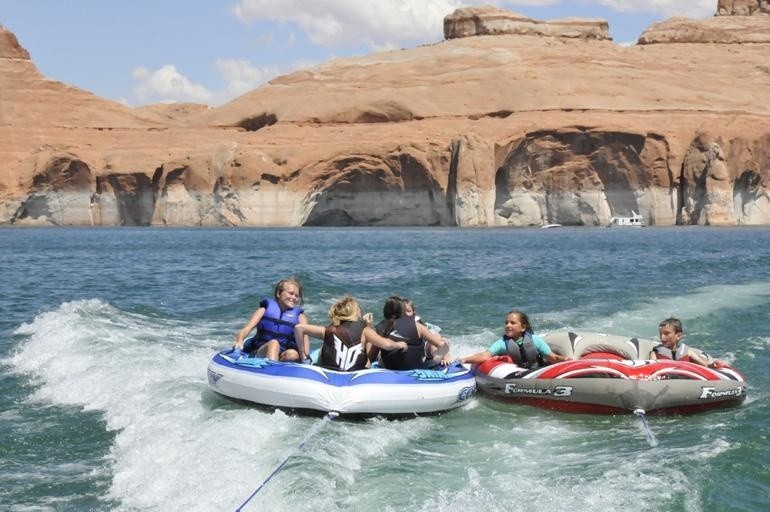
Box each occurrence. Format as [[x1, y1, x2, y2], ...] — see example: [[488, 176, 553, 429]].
[[208, 347, 476, 416], [472, 332, 748, 417], [601, 210, 648, 228]]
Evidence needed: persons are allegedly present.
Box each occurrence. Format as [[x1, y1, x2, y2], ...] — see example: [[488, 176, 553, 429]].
[[401, 296, 452, 364], [369, 296, 449, 370], [295, 296, 408, 373], [454, 311, 573, 367], [646, 317, 712, 367], [232, 278, 310, 364]]
